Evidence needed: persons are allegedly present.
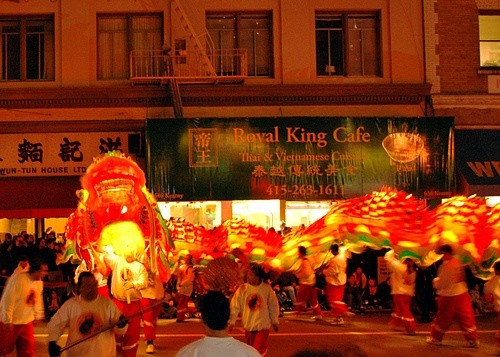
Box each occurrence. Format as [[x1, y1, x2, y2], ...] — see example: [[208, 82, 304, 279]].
[[226, 262, 279, 357], [169, 290, 262, 357], [384, 249, 417, 335], [270, 271, 300, 316], [288, 246, 322, 320], [140, 272, 164, 354], [469, 284, 482, 315], [159, 276, 178, 319], [484, 261, 500, 312], [47, 271, 129, 357], [0, 231, 81, 321], [104, 251, 148, 357], [347, 267, 392, 314], [320, 244, 347, 326], [426, 246, 481, 347], [176, 254, 195, 323], [0, 260, 48, 357]]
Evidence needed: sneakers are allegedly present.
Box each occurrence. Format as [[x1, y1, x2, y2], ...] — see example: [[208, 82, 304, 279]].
[[464, 338, 480, 346], [404, 329, 416, 334], [425, 335, 443, 345], [146, 344, 154, 353]]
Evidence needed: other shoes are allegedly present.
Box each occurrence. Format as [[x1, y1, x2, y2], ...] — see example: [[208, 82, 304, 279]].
[[176, 319, 184, 321]]
